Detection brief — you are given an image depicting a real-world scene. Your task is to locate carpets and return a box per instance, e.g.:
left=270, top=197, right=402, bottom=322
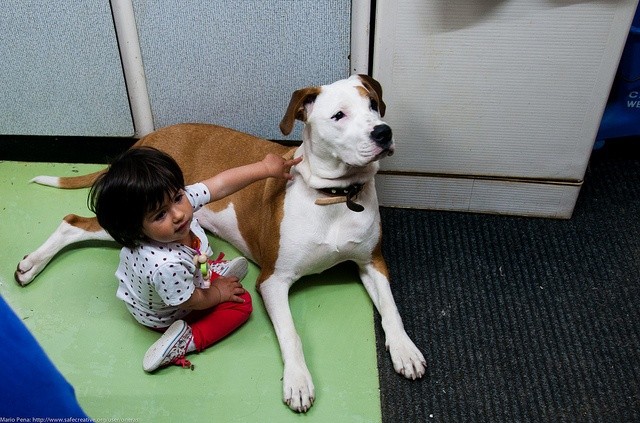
left=373, top=134, right=640, bottom=423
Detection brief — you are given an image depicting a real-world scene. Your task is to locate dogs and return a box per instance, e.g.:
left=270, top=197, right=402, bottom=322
left=12, top=72, right=431, bottom=416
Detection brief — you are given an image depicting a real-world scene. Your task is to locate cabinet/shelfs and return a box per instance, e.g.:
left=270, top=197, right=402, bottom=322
left=363, top=0, right=638, bottom=222
left=0, top=0, right=359, bottom=153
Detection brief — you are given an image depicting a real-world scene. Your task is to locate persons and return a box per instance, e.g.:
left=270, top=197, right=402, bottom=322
left=86, top=144, right=305, bottom=371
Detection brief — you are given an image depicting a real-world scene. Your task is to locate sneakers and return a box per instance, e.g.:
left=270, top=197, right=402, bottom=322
left=208, top=252, right=249, bottom=283
left=143, top=320, right=193, bottom=372
